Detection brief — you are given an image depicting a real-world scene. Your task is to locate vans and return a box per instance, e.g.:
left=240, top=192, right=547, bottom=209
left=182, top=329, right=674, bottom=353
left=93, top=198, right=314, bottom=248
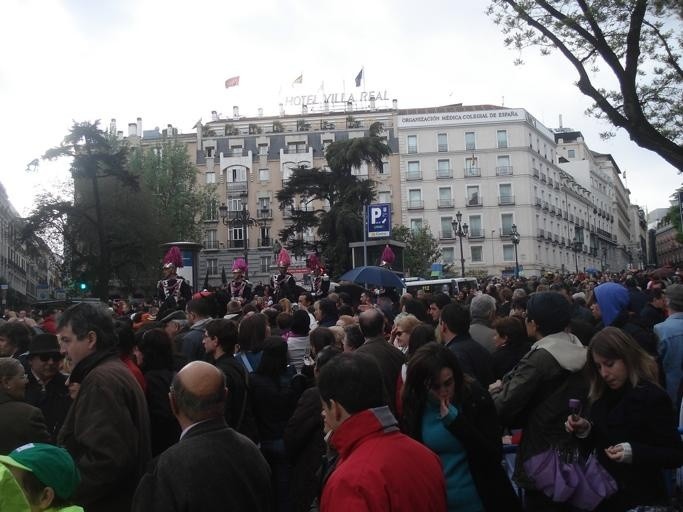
left=402, top=278, right=479, bottom=302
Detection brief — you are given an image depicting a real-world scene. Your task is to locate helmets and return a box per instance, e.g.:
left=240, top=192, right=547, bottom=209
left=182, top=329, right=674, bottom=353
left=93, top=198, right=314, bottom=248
left=379, top=261, right=392, bottom=268
left=162, top=261, right=176, bottom=275
left=277, top=261, right=288, bottom=270
left=232, top=267, right=244, bottom=275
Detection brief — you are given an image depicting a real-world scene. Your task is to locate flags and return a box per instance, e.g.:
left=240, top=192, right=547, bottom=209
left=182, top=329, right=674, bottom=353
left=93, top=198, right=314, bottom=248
left=292, top=75, right=302, bottom=84
left=354, top=69, right=362, bottom=88
left=224, top=76, right=239, bottom=89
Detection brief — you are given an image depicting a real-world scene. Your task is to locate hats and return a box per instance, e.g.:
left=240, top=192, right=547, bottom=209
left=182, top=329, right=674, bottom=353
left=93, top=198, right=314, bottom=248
left=156, top=309, right=188, bottom=326
left=139, top=328, right=176, bottom=368
left=24, top=334, right=63, bottom=364
left=0, top=442, right=83, bottom=502
left=661, top=283, right=683, bottom=306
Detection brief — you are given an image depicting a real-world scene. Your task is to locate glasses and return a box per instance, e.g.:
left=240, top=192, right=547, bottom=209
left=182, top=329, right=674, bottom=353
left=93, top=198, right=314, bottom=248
left=5, top=372, right=29, bottom=381
left=393, top=327, right=409, bottom=338
left=33, top=351, right=65, bottom=364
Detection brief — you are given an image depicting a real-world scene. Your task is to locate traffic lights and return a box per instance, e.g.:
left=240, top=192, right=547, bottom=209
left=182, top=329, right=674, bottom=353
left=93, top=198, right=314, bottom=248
left=80, top=272, right=88, bottom=292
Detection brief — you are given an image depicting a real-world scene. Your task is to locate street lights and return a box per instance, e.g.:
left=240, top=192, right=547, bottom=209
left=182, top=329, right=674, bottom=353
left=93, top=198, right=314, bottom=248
left=570, top=238, right=582, bottom=275
left=219, top=190, right=269, bottom=280
left=452, top=210, right=469, bottom=278
left=491, top=228, right=495, bottom=275
left=510, top=223, right=520, bottom=278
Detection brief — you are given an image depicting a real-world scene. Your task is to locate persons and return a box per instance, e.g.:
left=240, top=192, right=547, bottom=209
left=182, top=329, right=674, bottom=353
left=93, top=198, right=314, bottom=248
left=2, top=242, right=683, bottom=510
